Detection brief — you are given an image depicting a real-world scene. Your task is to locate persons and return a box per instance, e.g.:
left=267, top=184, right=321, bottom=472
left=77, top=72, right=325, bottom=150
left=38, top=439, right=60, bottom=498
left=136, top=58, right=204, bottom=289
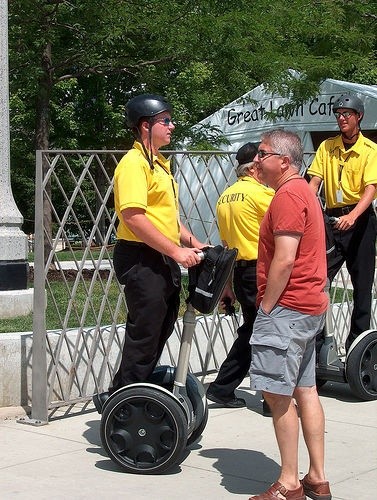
left=247, top=129, right=332, bottom=500
left=205, top=142, right=298, bottom=417
left=308, top=95, right=377, bottom=357
left=109, top=94, right=216, bottom=397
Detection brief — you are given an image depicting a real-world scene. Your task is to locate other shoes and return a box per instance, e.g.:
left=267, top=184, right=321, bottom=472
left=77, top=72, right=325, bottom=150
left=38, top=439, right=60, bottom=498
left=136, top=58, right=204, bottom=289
left=262, top=402, right=301, bottom=417
left=205, top=392, right=246, bottom=408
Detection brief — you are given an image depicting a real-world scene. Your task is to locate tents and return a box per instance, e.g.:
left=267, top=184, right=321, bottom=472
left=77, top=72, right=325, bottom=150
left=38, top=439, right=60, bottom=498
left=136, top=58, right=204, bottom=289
left=166, top=69, right=377, bottom=245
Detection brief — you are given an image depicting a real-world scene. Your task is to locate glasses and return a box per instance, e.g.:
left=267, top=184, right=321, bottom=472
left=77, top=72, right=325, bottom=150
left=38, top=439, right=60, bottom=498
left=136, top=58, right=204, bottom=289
left=334, top=112, right=357, bottom=118
left=255, top=151, right=282, bottom=158
left=153, top=117, right=176, bottom=126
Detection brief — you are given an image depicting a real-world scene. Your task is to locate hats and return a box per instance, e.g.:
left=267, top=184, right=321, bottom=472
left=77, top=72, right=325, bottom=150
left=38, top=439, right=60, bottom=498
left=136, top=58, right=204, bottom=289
left=236, top=142, right=262, bottom=164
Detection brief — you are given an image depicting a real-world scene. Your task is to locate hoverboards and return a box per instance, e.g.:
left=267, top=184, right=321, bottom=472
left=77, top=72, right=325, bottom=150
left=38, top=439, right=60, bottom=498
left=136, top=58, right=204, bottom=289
left=100, top=244, right=240, bottom=475
left=316, top=213, right=377, bottom=401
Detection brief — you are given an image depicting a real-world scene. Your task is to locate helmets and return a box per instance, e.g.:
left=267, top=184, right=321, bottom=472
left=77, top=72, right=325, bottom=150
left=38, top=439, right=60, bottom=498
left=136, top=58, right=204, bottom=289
left=333, top=95, right=364, bottom=114
left=125, top=94, right=174, bottom=127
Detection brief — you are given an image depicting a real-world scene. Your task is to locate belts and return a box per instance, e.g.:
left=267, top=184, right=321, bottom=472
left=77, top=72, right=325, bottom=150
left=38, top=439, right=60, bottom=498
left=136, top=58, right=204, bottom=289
left=325, top=203, right=358, bottom=217
left=234, top=261, right=257, bottom=267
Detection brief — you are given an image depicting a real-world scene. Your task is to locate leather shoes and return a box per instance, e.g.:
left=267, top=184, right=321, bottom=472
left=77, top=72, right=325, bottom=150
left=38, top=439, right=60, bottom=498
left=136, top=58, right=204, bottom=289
left=300, top=473, right=332, bottom=500
left=249, top=481, right=307, bottom=500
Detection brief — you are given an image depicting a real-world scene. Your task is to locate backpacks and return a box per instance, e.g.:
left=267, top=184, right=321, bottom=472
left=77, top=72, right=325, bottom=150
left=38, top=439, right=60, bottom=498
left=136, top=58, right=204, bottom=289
left=185, top=245, right=238, bottom=315
